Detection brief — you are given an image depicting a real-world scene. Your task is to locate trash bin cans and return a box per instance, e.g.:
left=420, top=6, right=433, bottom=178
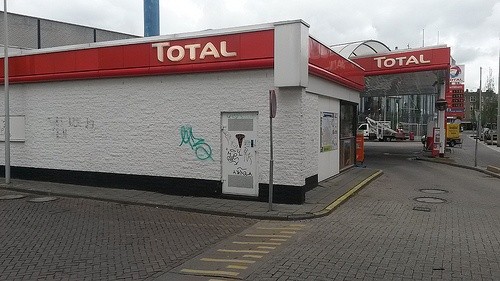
left=409, top=132, right=414, bottom=141
left=426, top=136, right=433, bottom=151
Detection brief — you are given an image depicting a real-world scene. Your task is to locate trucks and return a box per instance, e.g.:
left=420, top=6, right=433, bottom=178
left=358, top=123, right=409, bottom=142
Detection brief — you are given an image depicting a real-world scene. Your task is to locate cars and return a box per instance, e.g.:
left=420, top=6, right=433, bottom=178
left=421, top=126, right=499, bottom=148
